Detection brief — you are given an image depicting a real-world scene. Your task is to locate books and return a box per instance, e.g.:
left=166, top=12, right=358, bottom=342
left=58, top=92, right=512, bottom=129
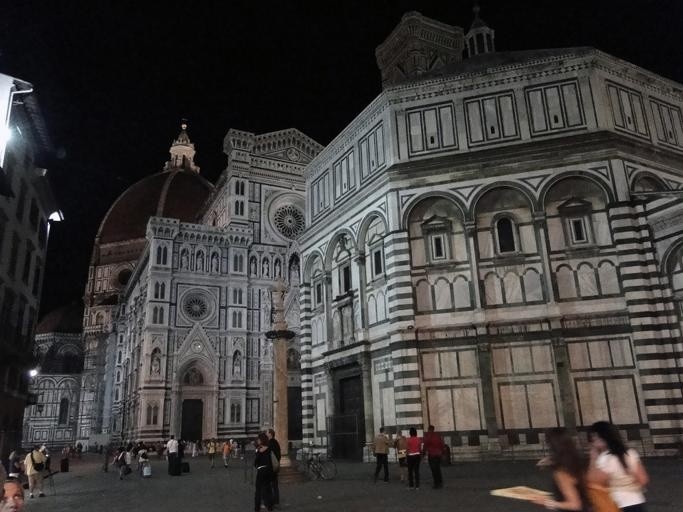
left=489, top=485, right=553, bottom=500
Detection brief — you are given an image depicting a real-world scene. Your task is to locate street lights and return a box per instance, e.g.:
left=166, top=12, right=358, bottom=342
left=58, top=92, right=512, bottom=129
left=33, top=404, right=45, bottom=445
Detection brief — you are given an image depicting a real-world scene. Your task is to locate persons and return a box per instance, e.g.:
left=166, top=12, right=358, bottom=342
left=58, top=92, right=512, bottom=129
left=0, top=427, right=282, bottom=511
left=393, top=429, right=407, bottom=481
left=587, top=421, right=651, bottom=512
left=404, top=426, right=422, bottom=489
left=535, top=425, right=587, bottom=512
left=372, top=428, right=395, bottom=482
left=422, top=424, right=445, bottom=489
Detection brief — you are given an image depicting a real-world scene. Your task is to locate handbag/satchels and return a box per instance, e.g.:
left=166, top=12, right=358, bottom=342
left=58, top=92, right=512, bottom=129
left=269, top=449, right=279, bottom=473
left=31, top=452, right=44, bottom=471
left=584, top=480, right=620, bottom=512
left=141, top=452, right=148, bottom=459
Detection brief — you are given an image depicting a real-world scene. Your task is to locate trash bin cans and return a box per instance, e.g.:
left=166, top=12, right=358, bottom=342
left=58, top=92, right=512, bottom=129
left=61, top=457, right=69, bottom=473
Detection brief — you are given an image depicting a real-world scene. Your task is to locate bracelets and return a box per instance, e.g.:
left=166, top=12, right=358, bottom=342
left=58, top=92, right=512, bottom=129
left=543, top=499, right=555, bottom=509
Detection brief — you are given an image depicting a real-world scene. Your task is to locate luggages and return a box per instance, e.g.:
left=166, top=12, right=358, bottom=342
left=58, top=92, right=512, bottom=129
left=141, top=463, right=152, bottom=478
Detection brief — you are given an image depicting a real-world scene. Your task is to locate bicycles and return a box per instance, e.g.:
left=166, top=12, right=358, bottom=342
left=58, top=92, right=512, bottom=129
left=296, top=452, right=337, bottom=480
